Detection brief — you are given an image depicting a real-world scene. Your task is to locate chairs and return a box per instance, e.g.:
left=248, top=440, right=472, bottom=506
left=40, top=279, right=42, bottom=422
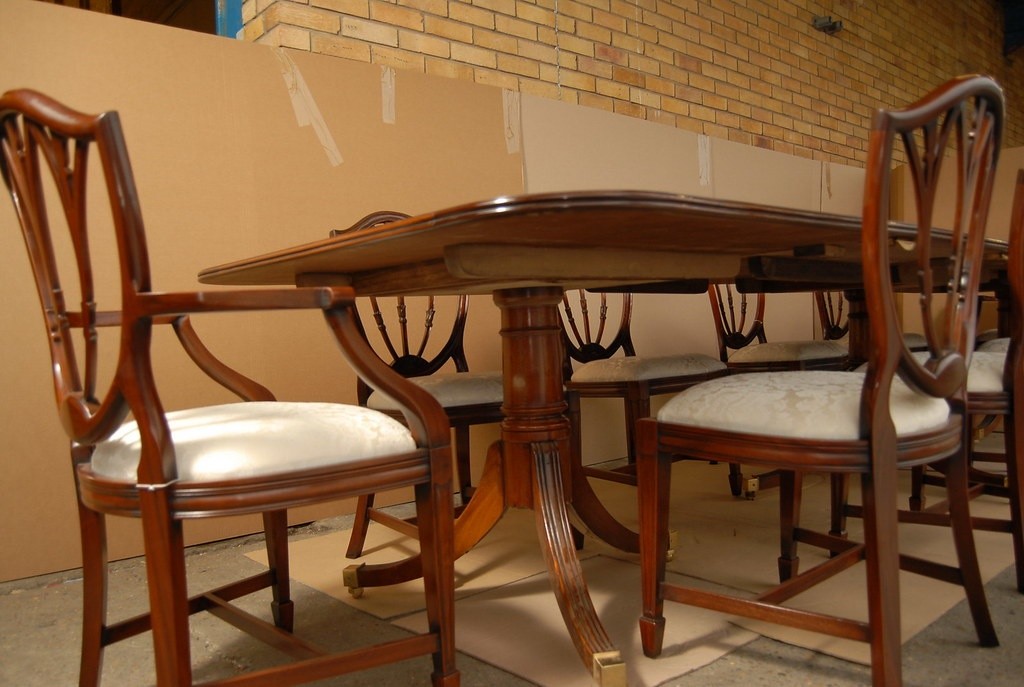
left=638, top=75, right=1006, bottom=686
left=558, top=284, right=743, bottom=495
left=2, top=90, right=463, bottom=686
left=829, top=170, right=1024, bottom=593
left=709, top=281, right=931, bottom=499
left=330, top=211, right=585, bottom=559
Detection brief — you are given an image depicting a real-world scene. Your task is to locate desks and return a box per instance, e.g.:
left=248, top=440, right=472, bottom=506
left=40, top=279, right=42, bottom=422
left=195, top=188, right=1008, bottom=687
